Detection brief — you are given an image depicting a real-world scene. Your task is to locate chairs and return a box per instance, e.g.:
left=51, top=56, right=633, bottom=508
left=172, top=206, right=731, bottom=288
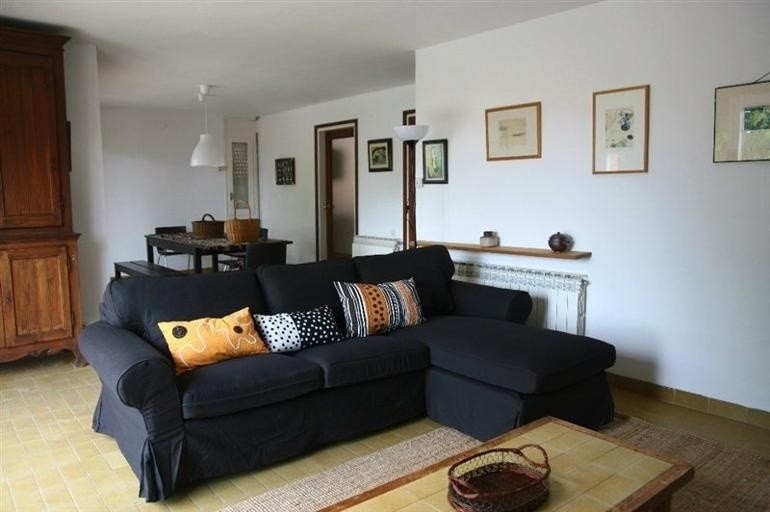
left=217, top=228, right=268, bottom=272
left=155, top=226, right=191, bottom=267
left=245, top=242, right=286, bottom=270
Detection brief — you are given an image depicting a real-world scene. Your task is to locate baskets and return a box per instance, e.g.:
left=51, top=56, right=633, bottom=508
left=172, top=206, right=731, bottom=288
left=191, top=213, right=225, bottom=239
left=225, top=202, right=260, bottom=243
left=447, top=444, right=551, bottom=512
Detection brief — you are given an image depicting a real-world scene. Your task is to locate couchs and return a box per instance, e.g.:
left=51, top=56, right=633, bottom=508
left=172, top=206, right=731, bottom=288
left=77, top=245, right=616, bottom=505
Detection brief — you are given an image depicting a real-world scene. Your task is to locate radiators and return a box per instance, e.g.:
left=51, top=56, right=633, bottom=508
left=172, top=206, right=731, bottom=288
left=350, top=236, right=401, bottom=258
left=450, top=261, right=589, bottom=337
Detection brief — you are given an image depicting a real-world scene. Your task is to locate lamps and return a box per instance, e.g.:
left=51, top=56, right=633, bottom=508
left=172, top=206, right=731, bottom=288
left=189, top=84, right=225, bottom=169
left=392, top=124, right=430, bottom=250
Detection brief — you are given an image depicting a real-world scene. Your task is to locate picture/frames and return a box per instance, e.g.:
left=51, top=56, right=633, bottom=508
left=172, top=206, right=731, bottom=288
left=713, top=80, right=770, bottom=163
left=592, top=84, right=650, bottom=175
left=485, top=101, right=542, bottom=161
left=367, top=137, right=393, bottom=172
left=422, top=139, right=448, bottom=184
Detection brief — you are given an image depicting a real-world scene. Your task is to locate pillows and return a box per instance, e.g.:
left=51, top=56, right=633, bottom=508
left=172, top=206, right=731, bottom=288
left=252, top=302, right=345, bottom=355
left=155, top=304, right=272, bottom=377
left=330, top=276, right=428, bottom=341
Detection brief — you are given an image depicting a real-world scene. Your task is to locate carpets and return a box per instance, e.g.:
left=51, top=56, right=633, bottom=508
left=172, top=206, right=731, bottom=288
left=214, top=408, right=770, bottom=512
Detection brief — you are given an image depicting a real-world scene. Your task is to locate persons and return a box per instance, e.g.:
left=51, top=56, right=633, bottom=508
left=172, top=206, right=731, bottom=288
left=372, top=147, right=386, bottom=165
left=427, top=146, right=443, bottom=179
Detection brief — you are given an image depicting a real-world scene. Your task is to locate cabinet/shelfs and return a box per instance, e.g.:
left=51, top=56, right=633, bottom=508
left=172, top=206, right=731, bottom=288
left=0, top=233, right=88, bottom=366
left=0, top=23, right=71, bottom=230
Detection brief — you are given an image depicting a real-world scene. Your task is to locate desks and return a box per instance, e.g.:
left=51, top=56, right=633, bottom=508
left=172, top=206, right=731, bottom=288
left=144, top=232, right=294, bottom=273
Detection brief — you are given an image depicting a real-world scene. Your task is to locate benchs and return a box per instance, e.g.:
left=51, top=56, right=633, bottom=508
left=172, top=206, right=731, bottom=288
left=112, top=260, right=189, bottom=279
